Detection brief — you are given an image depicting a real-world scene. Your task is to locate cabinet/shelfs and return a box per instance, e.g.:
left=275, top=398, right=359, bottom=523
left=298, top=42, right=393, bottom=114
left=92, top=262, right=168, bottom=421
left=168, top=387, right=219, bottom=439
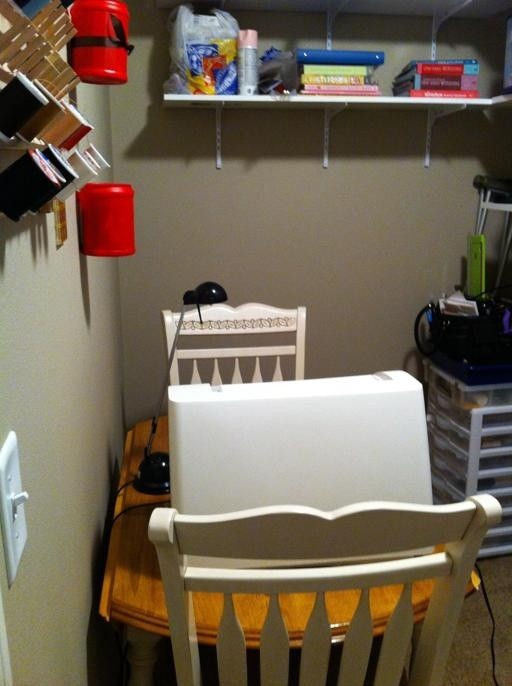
left=162, top=0, right=512, bottom=168
left=422, top=358, right=512, bottom=559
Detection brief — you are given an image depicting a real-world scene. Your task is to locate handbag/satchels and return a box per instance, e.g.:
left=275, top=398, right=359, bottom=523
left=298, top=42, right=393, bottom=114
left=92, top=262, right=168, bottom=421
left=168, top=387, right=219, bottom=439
left=414, top=287, right=511, bottom=365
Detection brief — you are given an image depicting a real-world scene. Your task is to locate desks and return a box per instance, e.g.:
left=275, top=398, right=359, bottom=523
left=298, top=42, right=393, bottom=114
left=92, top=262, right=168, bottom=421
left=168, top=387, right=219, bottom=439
left=99, top=415, right=475, bottom=686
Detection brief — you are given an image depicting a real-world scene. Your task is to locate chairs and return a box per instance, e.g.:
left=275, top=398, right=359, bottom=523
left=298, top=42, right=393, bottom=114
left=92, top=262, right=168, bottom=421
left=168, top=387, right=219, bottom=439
left=160, top=302, right=306, bottom=385
left=149, top=494, right=502, bottom=686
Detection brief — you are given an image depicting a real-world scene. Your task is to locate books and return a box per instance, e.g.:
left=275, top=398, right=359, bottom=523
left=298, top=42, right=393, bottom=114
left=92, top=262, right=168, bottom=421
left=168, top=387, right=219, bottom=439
left=392, top=58, right=480, bottom=97
left=443, top=297, right=478, bottom=315
left=294, top=48, right=385, bottom=96
